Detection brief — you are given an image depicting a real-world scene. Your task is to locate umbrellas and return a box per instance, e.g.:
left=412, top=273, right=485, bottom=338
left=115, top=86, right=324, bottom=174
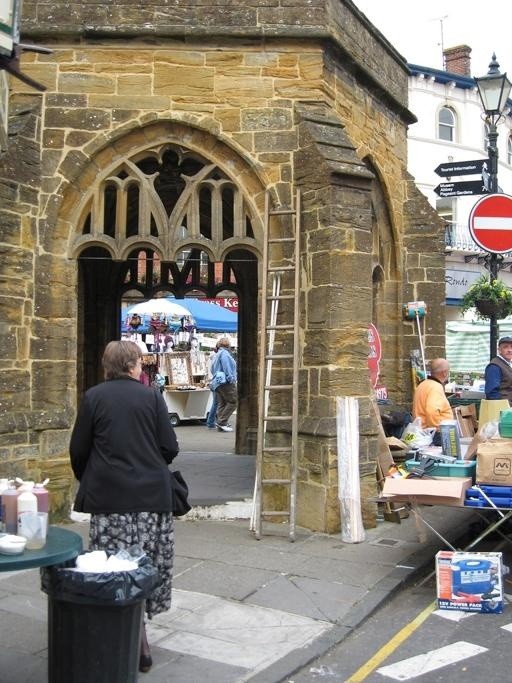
left=127, top=299, right=192, bottom=317
left=121, top=297, right=238, bottom=341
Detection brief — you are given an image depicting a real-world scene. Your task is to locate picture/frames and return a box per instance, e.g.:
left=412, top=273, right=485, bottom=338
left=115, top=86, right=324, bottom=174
left=166, top=350, right=195, bottom=385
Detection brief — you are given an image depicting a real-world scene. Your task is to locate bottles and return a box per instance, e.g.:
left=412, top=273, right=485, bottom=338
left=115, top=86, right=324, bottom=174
left=0, top=476, right=51, bottom=536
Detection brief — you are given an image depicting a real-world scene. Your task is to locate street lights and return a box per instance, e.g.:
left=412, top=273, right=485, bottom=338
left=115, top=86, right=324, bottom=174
left=471, top=48, right=512, bottom=359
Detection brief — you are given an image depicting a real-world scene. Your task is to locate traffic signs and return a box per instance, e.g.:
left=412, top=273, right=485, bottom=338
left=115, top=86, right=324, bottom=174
left=433, top=158, right=489, bottom=174
left=433, top=180, right=487, bottom=196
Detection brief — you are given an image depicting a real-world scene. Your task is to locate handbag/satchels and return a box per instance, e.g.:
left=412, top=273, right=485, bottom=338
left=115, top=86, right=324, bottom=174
left=173, top=471, right=191, bottom=516
left=215, top=370, right=227, bottom=384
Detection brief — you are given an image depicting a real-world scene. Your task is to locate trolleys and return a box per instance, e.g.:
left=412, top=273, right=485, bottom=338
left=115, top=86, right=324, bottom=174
left=159, top=386, right=215, bottom=427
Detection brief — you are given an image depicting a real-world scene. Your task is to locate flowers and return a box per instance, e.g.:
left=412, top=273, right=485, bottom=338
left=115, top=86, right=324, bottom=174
left=458, top=273, right=512, bottom=320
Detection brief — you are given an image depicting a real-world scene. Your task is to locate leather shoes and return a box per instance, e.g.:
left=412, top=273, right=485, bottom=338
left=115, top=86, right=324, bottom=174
left=140, top=655, right=152, bottom=672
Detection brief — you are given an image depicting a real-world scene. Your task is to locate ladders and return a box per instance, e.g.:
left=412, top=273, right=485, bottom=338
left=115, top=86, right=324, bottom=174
left=254, top=188, right=300, bottom=540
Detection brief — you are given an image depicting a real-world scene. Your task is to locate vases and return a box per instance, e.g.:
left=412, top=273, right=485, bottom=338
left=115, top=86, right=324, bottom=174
left=474, top=295, right=506, bottom=315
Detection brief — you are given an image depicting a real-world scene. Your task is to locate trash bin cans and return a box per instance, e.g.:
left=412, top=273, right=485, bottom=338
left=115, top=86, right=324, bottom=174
left=39, top=550, right=154, bottom=683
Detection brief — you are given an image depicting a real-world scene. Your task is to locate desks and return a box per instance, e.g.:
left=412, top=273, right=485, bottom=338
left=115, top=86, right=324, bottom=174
left=0, top=521, right=83, bottom=576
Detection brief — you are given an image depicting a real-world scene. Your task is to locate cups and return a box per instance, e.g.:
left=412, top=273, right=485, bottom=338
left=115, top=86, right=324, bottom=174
left=1, top=534, right=26, bottom=555
left=200, top=380, right=205, bottom=387
left=19, top=511, right=46, bottom=550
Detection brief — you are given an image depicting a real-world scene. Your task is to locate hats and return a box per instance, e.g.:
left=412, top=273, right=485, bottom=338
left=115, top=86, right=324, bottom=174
left=499, top=337, right=512, bottom=344
left=189, top=337, right=198, bottom=348
left=164, top=336, right=173, bottom=345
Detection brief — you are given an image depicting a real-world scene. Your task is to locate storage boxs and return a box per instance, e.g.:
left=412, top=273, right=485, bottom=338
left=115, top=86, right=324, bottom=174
left=379, top=474, right=475, bottom=508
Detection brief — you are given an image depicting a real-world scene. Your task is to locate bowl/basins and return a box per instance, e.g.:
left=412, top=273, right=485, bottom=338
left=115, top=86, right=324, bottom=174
left=164, top=385, right=178, bottom=389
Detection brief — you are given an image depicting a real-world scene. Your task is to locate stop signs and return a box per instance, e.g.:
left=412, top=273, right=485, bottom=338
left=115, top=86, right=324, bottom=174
left=468, top=194, right=511, bottom=255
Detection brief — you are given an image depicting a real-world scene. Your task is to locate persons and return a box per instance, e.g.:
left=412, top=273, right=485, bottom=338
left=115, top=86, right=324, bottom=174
left=164, top=335, right=175, bottom=353
left=207, top=341, right=222, bottom=429
left=483, top=336, right=511, bottom=407
left=210, top=337, right=238, bottom=432
left=69, top=339, right=181, bottom=673
left=189, top=335, right=200, bottom=352
left=413, top=356, right=454, bottom=445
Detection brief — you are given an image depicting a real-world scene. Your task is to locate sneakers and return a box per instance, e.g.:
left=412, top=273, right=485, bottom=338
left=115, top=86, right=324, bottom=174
left=208, top=423, right=234, bottom=432
